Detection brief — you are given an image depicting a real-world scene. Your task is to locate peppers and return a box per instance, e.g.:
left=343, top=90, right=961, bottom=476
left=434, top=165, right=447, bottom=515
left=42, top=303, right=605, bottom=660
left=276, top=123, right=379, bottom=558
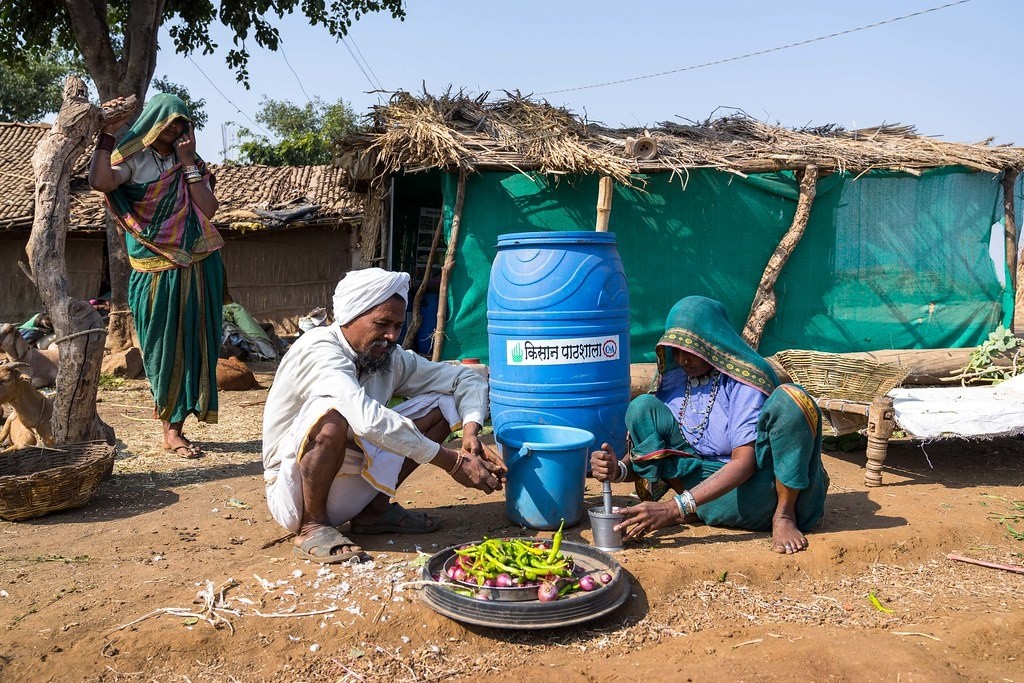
left=450, top=518, right=580, bottom=599
left=718, top=570, right=727, bottom=583
left=869, top=592, right=896, bottom=613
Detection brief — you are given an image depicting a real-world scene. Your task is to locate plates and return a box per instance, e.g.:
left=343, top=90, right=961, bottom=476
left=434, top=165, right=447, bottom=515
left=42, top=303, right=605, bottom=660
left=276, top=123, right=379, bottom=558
left=422, top=537, right=630, bottom=629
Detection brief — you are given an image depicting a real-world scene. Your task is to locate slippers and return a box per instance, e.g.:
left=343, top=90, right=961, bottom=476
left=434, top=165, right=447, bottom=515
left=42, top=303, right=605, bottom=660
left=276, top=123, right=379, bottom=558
left=293, top=526, right=364, bottom=563
left=350, top=502, right=441, bottom=534
left=164, top=443, right=202, bottom=459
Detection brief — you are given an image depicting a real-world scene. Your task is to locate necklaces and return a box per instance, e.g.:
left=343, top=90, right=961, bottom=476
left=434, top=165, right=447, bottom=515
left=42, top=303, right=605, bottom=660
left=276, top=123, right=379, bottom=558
left=678, top=370, right=720, bottom=445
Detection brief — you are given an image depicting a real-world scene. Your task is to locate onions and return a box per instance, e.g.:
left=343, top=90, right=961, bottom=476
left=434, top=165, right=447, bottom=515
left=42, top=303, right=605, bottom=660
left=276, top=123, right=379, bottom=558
left=430, top=537, right=612, bottom=602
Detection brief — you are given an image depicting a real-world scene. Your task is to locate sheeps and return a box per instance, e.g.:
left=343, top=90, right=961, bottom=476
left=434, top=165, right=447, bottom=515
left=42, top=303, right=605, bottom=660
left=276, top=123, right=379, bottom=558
left=214, top=355, right=269, bottom=390
left=0, top=322, right=60, bottom=453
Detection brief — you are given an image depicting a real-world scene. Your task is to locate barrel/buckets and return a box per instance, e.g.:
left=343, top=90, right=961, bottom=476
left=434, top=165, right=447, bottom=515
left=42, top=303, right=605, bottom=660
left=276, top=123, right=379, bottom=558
left=486, top=232, right=631, bottom=530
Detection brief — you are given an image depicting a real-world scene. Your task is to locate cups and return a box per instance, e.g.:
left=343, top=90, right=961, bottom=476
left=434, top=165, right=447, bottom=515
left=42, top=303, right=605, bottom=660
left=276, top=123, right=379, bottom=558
left=588, top=506, right=626, bottom=551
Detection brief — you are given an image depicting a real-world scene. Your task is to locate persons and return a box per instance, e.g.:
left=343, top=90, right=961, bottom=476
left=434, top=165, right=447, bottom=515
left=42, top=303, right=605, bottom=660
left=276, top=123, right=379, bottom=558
left=17, top=312, right=51, bottom=343
left=263, top=267, right=509, bottom=564
left=88, top=92, right=234, bottom=458
left=589, top=295, right=830, bottom=555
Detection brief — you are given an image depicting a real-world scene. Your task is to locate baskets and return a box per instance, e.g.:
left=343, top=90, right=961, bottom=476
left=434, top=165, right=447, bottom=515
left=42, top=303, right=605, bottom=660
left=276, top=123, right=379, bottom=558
left=0, top=439, right=118, bottom=521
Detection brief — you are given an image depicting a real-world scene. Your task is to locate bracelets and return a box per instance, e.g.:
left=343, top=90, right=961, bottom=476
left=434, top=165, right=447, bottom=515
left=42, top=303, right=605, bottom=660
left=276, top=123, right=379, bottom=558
left=95, top=131, right=115, bottom=154
left=614, top=461, right=628, bottom=483
left=183, top=165, right=202, bottom=184
left=675, top=489, right=696, bottom=519
left=446, top=450, right=463, bottom=475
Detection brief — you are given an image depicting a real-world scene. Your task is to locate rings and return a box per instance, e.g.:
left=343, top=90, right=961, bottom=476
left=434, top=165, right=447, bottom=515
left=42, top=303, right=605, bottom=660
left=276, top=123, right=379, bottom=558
left=179, top=138, right=184, bottom=142
